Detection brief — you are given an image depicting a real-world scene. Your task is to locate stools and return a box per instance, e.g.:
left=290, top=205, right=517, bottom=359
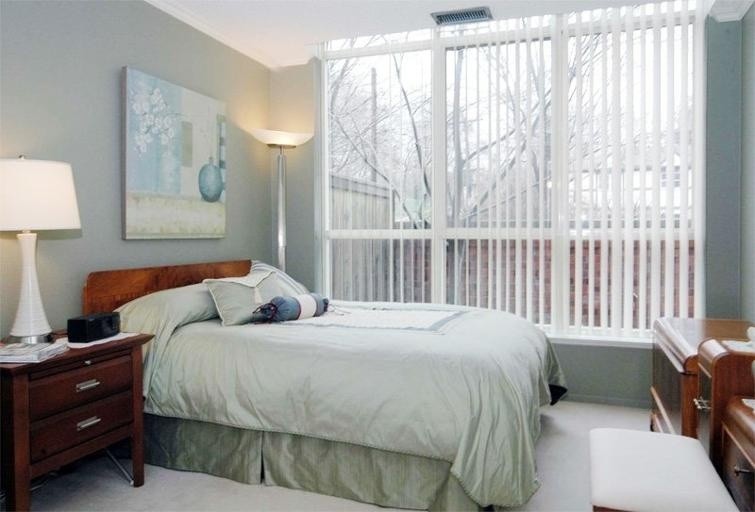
left=588, top=429, right=739, bottom=512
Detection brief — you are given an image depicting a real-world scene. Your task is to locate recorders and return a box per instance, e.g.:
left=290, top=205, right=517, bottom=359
left=66, top=311, right=120, bottom=343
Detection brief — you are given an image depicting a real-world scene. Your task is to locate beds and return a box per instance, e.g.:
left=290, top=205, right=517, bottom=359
left=84, top=260, right=555, bottom=512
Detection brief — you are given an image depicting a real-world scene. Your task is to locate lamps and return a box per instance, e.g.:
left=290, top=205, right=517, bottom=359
left=1, top=155, right=83, bottom=344
left=246, top=127, right=314, bottom=273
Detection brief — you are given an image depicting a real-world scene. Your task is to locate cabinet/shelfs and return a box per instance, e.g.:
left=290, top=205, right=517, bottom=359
left=0, top=328, right=155, bottom=511
left=650, top=317, right=755, bottom=511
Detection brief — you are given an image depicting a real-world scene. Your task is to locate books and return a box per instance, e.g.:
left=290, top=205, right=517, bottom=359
left=0, top=343, right=68, bottom=364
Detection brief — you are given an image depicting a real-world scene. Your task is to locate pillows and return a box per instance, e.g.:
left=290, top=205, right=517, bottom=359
left=201, top=271, right=328, bottom=325
